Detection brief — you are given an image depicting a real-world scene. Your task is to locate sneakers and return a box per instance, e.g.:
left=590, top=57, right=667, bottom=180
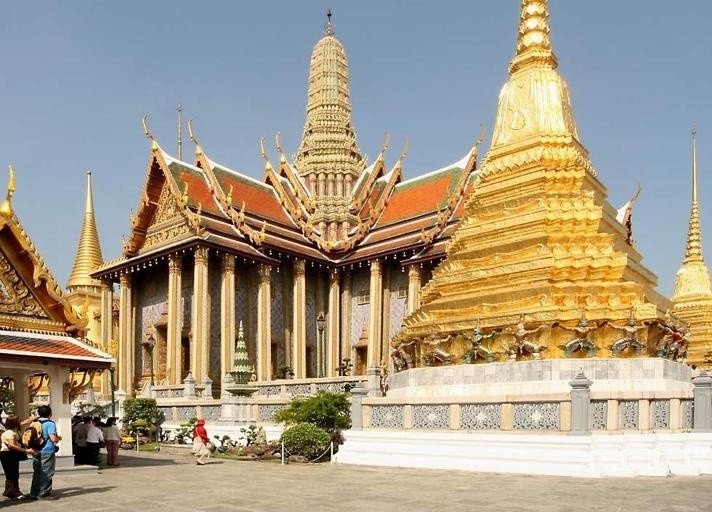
left=38, top=492, right=59, bottom=501
left=195, top=459, right=204, bottom=464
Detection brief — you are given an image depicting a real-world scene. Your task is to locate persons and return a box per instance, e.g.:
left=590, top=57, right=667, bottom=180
left=376, top=356, right=391, bottom=398
left=460, top=328, right=496, bottom=363
left=668, top=318, right=687, bottom=358
left=391, top=335, right=415, bottom=370
left=608, top=318, right=646, bottom=348
left=505, top=322, right=548, bottom=357
left=0, top=414, right=41, bottom=500
left=553, top=314, right=608, bottom=354
left=656, top=312, right=678, bottom=356
left=676, top=320, right=693, bottom=358
left=71, top=414, right=124, bottom=469
left=22, top=404, right=63, bottom=502
left=414, top=333, right=452, bottom=365
left=190, top=418, right=212, bottom=465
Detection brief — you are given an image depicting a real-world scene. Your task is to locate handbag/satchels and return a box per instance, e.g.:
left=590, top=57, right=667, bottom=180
left=206, top=443, right=213, bottom=451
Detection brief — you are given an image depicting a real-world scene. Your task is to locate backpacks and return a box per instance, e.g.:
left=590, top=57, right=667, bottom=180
left=22, top=419, right=52, bottom=451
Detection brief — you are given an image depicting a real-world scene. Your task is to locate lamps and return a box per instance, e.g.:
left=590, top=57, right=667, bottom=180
left=316, top=311, right=326, bottom=334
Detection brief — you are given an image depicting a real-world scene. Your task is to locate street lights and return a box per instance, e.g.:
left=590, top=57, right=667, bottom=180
left=147, top=334, right=156, bottom=385
left=107, top=358, right=116, bottom=418
left=316, top=313, right=328, bottom=377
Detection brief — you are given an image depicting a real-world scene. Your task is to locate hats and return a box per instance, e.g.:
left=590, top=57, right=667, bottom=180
left=197, top=419, right=205, bottom=425
